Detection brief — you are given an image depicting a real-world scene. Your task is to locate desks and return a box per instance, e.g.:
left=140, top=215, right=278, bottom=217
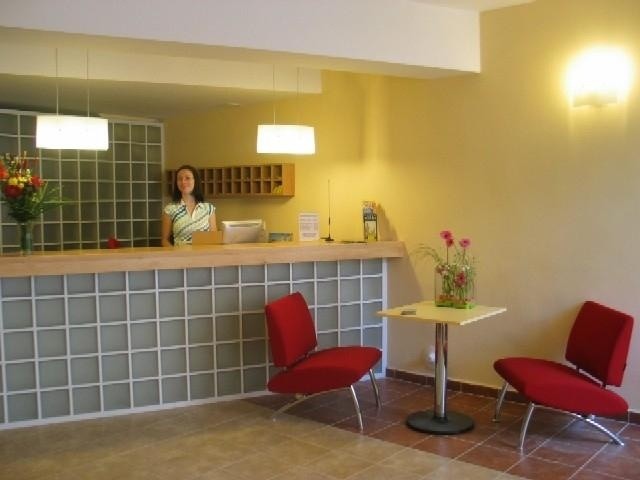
left=377, top=299, right=507, bottom=437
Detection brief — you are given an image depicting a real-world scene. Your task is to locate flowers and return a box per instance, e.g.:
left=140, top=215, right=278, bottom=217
left=455, top=238, right=485, bottom=271
left=0, top=151, right=70, bottom=249
left=418, top=229, right=455, bottom=268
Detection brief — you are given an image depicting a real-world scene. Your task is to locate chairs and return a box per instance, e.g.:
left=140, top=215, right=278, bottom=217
left=261, top=291, right=380, bottom=434
left=490, top=302, right=635, bottom=452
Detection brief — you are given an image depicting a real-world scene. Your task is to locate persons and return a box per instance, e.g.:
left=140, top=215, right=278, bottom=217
left=160, top=164, right=218, bottom=247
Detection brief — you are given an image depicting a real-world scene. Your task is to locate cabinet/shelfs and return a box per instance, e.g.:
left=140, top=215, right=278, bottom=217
left=165, top=162, right=294, bottom=198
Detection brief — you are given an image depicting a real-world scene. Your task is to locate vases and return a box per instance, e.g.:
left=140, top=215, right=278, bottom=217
left=454, top=271, right=476, bottom=309
left=18, top=217, right=35, bottom=255
left=434, top=270, right=454, bottom=307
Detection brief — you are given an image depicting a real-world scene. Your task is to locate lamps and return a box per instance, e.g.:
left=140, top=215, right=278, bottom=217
left=35, top=46, right=109, bottom=152
left=255, top=63, right=317, bottom=155
